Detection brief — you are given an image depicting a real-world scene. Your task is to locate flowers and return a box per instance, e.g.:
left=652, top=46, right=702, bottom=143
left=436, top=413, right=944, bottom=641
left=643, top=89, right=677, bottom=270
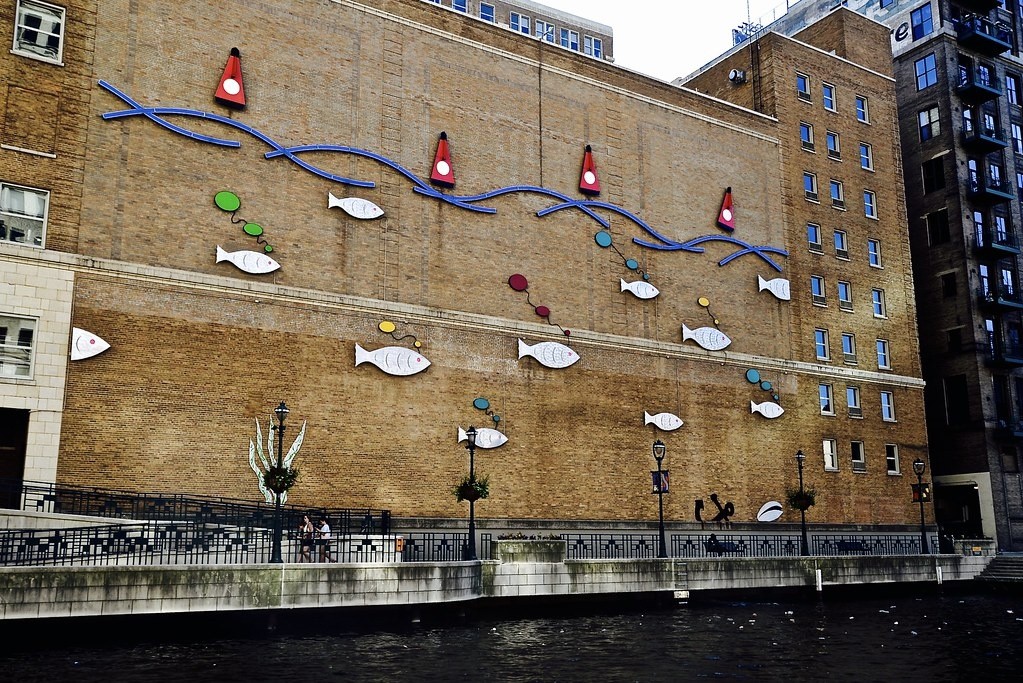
left=785, top=481, right=816, bottom=511
left=454, top=470, right=490, bottom=503
left=262, top=465, right=299, bottom=493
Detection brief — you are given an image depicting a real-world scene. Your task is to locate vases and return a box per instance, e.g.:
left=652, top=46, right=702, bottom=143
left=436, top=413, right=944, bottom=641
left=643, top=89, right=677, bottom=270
left=463, top=488, right=482, bottom=502
left=793, top=498, right=811, bottom=511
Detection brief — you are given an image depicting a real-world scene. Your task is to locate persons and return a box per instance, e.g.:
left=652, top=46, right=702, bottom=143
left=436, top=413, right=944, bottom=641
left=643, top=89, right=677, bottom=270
left=297, top=515, right=314, bottom=563
left=708, top=534, right=724, bottom=557
left=315, top=517, right=336, bottom=563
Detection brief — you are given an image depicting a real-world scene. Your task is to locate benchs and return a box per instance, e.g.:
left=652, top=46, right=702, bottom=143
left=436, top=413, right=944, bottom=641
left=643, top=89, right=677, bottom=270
left=836, top=542, right=872, bottom=555
left=704, top=541, right=743, bottom=557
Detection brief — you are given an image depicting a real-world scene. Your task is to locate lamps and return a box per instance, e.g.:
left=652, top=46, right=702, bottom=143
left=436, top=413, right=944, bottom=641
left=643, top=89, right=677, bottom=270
left=729, top=68, right=747, bottom=85
